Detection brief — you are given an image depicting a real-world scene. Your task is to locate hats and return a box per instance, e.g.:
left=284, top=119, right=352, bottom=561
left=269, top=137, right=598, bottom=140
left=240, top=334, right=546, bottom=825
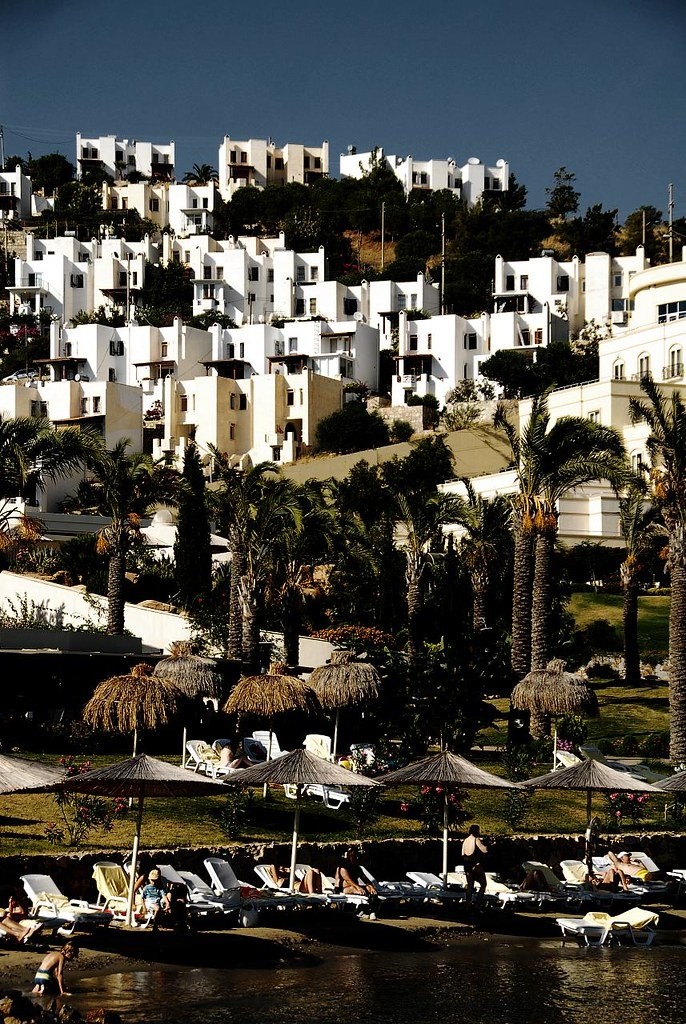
left=148, top=870, right=158, bottom=879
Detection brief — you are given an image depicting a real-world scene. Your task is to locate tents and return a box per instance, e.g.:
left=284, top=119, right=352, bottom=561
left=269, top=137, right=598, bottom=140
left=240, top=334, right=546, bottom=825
left=62, top=753, right=237, bottom=933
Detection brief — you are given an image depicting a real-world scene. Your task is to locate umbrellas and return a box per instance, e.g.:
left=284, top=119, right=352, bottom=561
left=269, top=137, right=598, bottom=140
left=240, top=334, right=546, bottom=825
left=224, top=747, right=385, bottom=897
left=519, top=758, right=668, bottom=881
left=650, top=768, right=686, bottom=796
left=375, top=749, right=527, bottom=891
left=510, top=658, right=600, bottom=774
left=84, top=640, right=381, bottom=807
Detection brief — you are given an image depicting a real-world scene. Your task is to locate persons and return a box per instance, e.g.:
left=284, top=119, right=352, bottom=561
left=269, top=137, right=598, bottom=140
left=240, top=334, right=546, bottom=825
left=218, top=738, right=272, bottom=769
left=278, top=846, right=378, bottom=900
left=607, top=850, right=686, bottom=888
left=0, top=879, right=34, bottom=944
left=460, top=825, right=497, bottom=909
left=131, top=852, right=173, bottom=933
left=31, top=940, right=80, bottom=996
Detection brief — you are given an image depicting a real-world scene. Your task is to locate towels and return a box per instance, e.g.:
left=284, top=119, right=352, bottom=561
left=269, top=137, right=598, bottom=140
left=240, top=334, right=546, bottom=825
left=583, top=906, right=660, bottom=930
left=303, top=735, right=331, bottom=761
left=92, top=865, right=130, bottom=901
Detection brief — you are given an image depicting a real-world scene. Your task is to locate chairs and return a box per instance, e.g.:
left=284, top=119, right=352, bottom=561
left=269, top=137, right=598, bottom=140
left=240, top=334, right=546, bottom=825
left=184, top=731, right=383, bottom=807
left=550, top=747, right=649, bottom=781
left=20, top=851, right=686, bottom=948
